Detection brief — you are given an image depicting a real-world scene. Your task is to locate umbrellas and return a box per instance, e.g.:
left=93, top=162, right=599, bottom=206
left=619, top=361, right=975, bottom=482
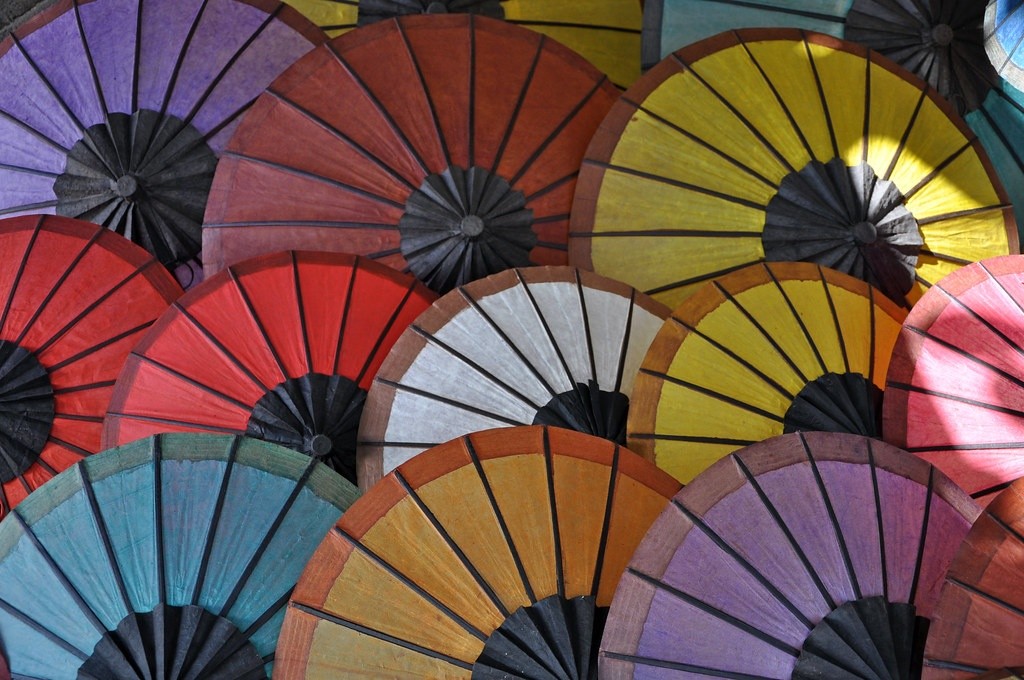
left=921, top=477, right=1024, bottom=680
left=627, top=262, right=909, bottom=484
left=641, top=0, right=1024, bottom=249
left=567, top=28, right=1020, bottom=314
left=285, top=0, right=642, bottom=94
left=0, top=431, right=362, bottom=680
left=101, top=249, right=438, bottom=486
left=0, top=213, right=185, bottom=521
left=355, top=264, right=671, bottom=492
left=597, top=432, right=983, bottom=680
left=203, top=12, right=617, bottom=293
left=883, top=254, right=1024, bottom=509
left=271, top=425, right=683, bottom=680
left=983, top=0, right=1024, bottom=92
left=0, top=0, right=330, bottom=290
